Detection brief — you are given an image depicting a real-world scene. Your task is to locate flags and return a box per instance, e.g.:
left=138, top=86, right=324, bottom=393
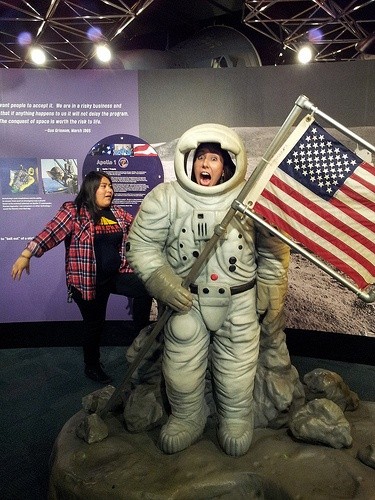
left=243, top=113, right=375, bottom=290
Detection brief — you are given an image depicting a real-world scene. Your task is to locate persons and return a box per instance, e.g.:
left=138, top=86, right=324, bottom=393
left=11, top=170, right=154, bottom=384
left=191, top=140, right=235, bottom=189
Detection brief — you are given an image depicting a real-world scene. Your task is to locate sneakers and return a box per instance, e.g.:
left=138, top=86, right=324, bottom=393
left=85, top=366, right=112, bottom=383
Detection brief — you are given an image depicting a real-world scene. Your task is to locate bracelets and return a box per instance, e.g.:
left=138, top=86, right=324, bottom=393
left=20, top=254, right=30, bottom=260
